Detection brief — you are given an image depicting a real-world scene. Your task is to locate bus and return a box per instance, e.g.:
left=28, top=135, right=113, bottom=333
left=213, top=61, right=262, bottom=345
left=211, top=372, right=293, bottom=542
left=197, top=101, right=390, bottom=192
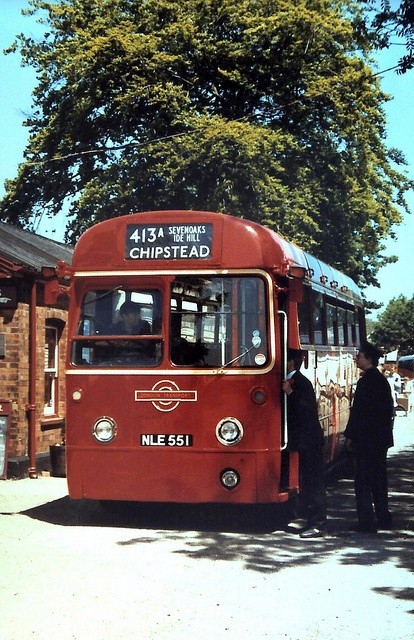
left=54, top=209, right=370, bottom=502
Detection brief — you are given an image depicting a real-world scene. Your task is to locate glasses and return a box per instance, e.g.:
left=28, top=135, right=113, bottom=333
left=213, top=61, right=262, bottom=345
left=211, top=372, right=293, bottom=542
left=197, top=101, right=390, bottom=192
left=356, top=355, right=360, bottom=358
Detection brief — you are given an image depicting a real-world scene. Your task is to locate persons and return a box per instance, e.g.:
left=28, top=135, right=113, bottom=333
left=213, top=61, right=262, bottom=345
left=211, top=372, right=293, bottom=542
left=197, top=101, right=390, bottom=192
left=390, top=361, right=400, bottom=417
left=381, top=369, right=390, bottom=378
left=284, top=350, right=335, bottom=537
left=408, top=375, right=413, bottom=422
left=96, top=301, right=155, bottom=365
left=343, top=343, right=394, bottom=532
left=404, top=377, right=407, bottom=389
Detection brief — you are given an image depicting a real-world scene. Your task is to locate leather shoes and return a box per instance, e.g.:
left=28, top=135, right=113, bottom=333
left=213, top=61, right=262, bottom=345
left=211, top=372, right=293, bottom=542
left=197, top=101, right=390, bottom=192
left=300, top=529, right=324, bottom=538
left=378, top=523, right=392, bottom=530
left=348, top=525, right=376, bottom=534
left=287, top=519, right=308, bottom=534
